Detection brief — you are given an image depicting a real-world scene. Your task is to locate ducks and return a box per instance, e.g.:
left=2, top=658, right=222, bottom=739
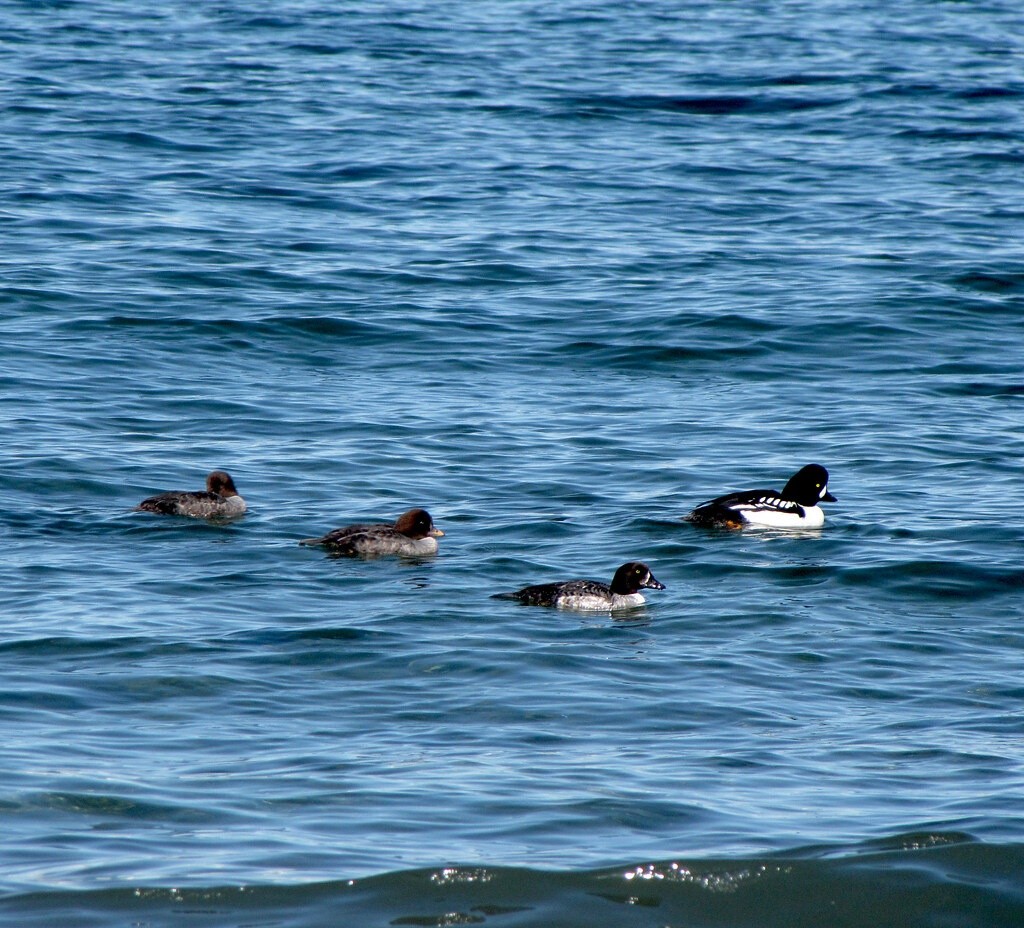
left=490, top=562, right=665, bottom=612
left=135, top=471, right=246, bottom=518
left=299, top=509, right=445, bottom=556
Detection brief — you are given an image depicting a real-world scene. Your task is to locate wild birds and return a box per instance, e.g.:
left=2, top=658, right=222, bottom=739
left=683, top=464, right=838, bottom=528
